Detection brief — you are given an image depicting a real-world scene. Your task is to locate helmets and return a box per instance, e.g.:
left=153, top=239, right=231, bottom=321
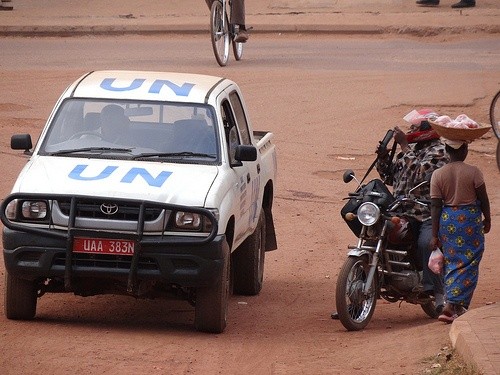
left=403, top=109, right=441, bottom=143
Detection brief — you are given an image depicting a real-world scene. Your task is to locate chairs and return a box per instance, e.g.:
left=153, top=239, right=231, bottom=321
left=83, top=112, right=100, bottom=137
left=173, top=119, right=207, bottom=153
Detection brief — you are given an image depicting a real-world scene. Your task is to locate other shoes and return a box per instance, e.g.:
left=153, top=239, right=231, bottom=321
left=215, top=20, right=223, bottom=41
left=451, top=0, right=476, bottom=7
left=331, top=303, right=364, bottom=319
left=416, top=0, right=439, bottom=4
left=434, top=294, right=445, bottom=312
left=235, top=30, right=248, bottom=43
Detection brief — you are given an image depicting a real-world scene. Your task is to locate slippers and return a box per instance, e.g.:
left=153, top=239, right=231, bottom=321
left=438, top=314, right=459, bottom=323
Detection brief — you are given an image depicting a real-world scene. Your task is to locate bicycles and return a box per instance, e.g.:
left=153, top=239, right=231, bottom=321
left=209, top=0, right=253, bottom=66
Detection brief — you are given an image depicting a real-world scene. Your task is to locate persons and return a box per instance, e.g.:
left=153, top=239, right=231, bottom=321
left=205, top=0, right=249, bottom=43
left=416, top=0, right=476, bottom=9
left=81, top=105, right=131, bottom=150
left=429, top=140, right=493, bottom=322
left=331, top=106, right=451, bottom=319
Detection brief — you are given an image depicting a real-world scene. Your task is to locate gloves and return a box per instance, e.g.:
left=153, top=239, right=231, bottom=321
left=394, top=126, right=408, bottom=147
left=374, top=140, right=392, bottom=157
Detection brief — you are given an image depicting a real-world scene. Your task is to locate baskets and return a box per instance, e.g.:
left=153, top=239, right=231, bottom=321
left=428, top=117, right=492, bottom=141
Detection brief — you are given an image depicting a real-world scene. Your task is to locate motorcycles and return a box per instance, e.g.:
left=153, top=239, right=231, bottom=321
left=335, top=171, right=436, bottom=330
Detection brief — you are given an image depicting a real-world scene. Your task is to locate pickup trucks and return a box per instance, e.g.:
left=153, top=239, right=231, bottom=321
left=0, top=70, right=278, bottom=333
